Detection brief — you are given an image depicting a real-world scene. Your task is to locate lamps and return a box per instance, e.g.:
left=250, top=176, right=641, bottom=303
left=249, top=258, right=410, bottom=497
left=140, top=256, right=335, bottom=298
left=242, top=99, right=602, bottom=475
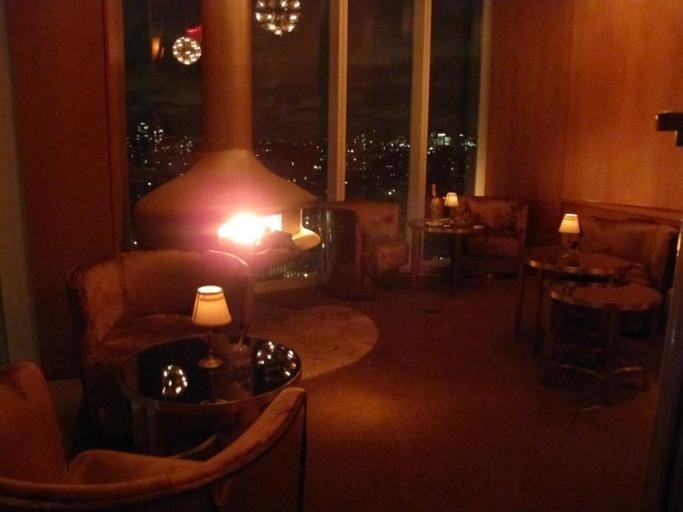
left=191, top=284, right=231, bottom=369
left=444, top=192, right=458, bottom=207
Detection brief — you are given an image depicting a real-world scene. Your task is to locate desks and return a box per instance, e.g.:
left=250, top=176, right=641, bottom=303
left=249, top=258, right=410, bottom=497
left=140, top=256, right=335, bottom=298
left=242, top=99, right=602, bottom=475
left=117, top=334, right=301, bottom=458
left=542, top=281, right=662, bottom=404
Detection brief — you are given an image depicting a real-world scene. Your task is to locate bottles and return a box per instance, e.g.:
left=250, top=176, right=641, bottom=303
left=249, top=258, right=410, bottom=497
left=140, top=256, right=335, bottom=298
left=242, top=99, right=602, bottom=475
left=430, top=182, right=442, bottom=224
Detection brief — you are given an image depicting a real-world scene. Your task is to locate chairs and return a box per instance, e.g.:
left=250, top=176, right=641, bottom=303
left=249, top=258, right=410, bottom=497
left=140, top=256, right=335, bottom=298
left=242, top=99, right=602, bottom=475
left=0, top=362, right=307, bottom=512
left=65, top=251, right=254, bottom=449
